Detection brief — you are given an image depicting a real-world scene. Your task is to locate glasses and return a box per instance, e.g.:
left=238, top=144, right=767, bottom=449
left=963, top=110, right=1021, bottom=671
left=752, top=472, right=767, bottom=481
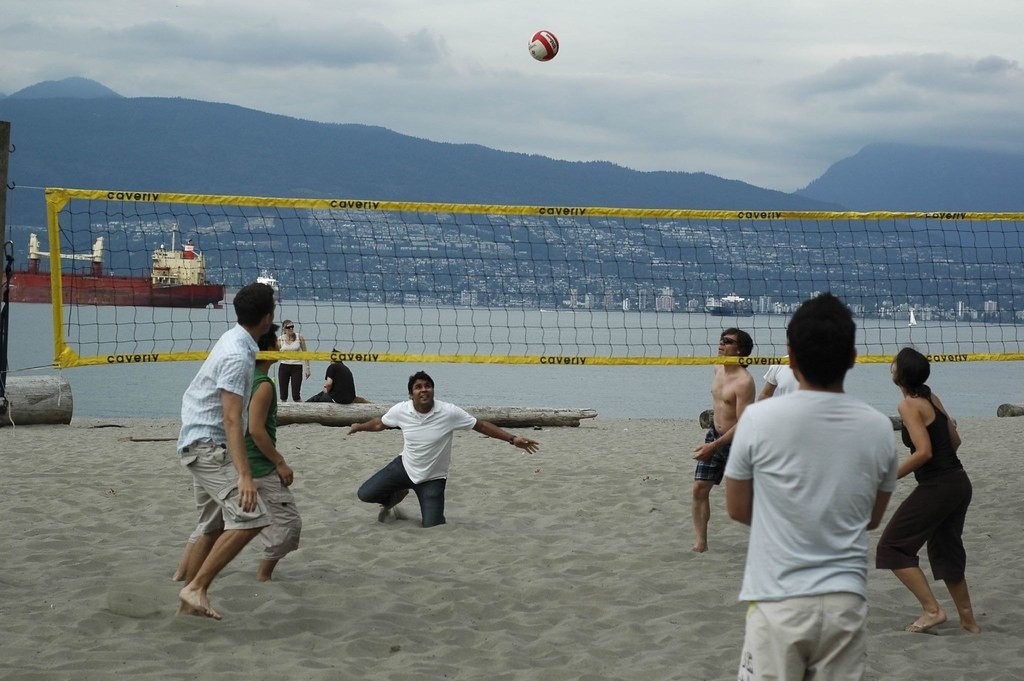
left=285, top=325, right=294, bottom=329
left=720, top=337, right=737, bottom=344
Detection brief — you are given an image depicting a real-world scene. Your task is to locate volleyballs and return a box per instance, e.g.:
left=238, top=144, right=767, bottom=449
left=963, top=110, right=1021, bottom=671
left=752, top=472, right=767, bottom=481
left=528, top=30, right=560, bottom=63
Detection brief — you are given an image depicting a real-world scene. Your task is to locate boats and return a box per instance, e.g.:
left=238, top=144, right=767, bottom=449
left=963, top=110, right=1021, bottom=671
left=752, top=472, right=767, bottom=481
left=257, top=269, right=285, bottom=303
left=0, top=221, right=227, bottom=309
left=705, top=293, right=755, bottom=317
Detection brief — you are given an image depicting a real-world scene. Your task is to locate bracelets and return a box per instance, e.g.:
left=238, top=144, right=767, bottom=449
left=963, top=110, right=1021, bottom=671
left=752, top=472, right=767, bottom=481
left=510, top=433, right=517, bottom=444
left=306, top=366, right=311, bottom=368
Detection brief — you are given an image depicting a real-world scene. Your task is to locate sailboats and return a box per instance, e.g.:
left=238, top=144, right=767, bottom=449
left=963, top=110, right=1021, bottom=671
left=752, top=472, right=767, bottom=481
left=908, top=309, right=917, bottom=326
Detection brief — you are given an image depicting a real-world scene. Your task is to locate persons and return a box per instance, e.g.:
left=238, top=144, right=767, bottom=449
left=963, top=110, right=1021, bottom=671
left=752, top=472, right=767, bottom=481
left=277, top=319, right=311, bottom=402
left=304, top=349, right=356, bottom=404
left=172, top=322, right=301, bottom=583
left=757, top=353, right=800, bottom=400
left=174, top=281, right=275, bottom=619
left=725, top=291, right=899, bottom=681
left=875, top=346, right=982, bottom=635
left=691, top=328, right=756, bottom=553
left=347, top=371, right=540, bottom=527
